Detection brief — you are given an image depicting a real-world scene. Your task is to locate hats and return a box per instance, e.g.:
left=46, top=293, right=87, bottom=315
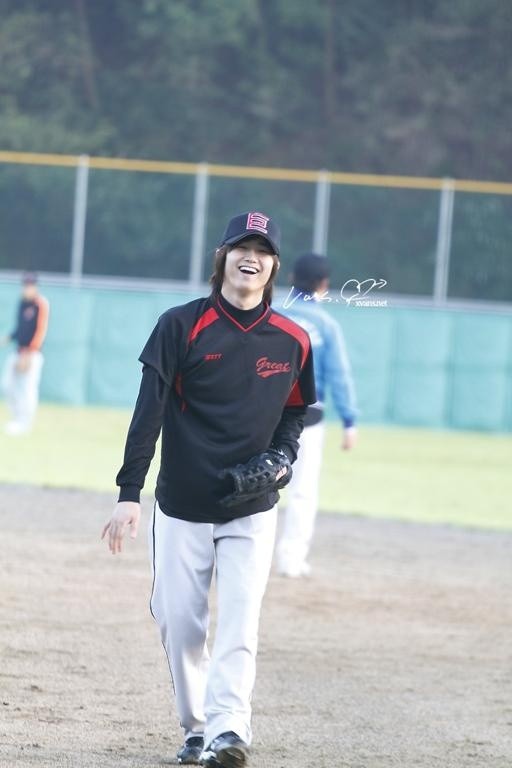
left=294, top=253, right=334, bottom=280
left=220, top=211, right=281, bottom=257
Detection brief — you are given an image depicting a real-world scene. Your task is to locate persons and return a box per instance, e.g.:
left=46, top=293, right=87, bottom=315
left=269, top=252, right=360, bottom=582
left=0, top=268, right=52, bottom=438
left=97, top=211, right=319, bottom=768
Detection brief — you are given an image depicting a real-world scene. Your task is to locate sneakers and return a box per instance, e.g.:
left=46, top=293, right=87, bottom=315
left=176, top=736, right=205, bottom=765
left=278, top=560, right=311, bottom=578
left=198, top=731, right=250, bottom=768
